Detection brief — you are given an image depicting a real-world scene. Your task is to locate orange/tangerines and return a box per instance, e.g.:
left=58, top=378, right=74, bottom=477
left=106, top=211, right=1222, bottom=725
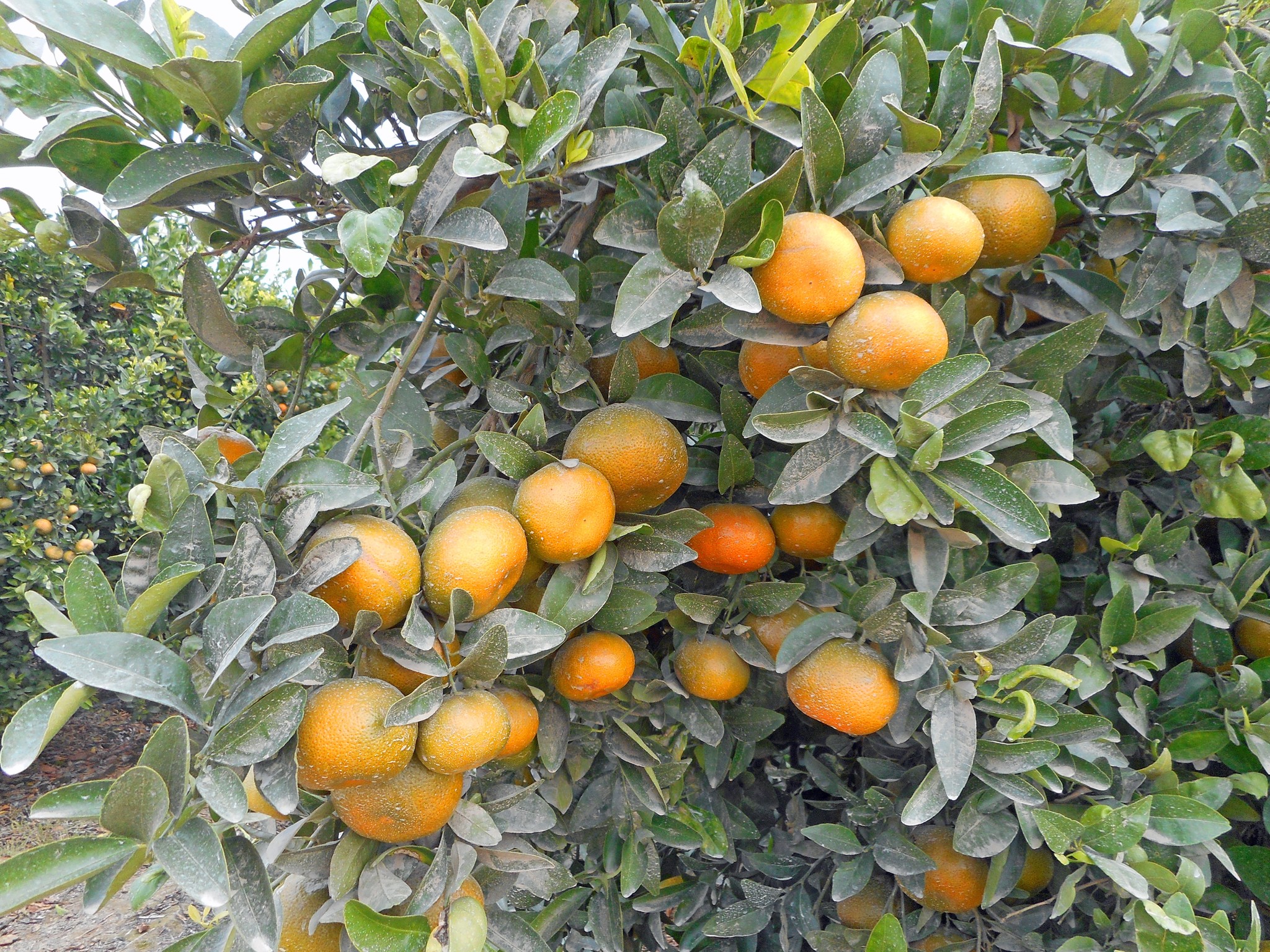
left=246, top=176, right=1270, bottom=952
left=4, top=218, right=343, bottom=563
